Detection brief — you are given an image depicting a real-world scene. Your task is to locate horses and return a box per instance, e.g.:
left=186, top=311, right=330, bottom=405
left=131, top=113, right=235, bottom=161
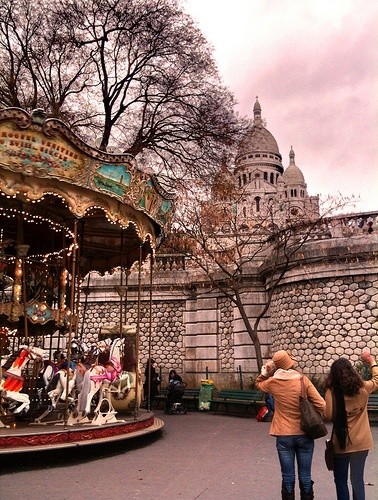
left=0, top=336, right=127, bottom=415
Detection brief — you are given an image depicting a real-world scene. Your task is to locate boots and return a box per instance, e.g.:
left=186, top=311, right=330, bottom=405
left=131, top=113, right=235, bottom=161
left=281, top=480, right=296, bottom=500
left=300, top=480, right=315, bottom=500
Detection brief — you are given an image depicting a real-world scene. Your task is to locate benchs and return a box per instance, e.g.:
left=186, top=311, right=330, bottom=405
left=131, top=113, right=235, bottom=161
left=367, top=392, right=378, bottom=412
left=154, top=387, right=201, bottom=410
left=207, top=388, right=264, bottom=416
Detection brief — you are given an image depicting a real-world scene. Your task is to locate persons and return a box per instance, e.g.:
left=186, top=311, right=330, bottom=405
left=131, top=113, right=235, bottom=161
left=165, top=370, right=184, bottom=413
left=255, top=350, right=327, bottom=500
left=144, top=360, right=161, bottom=411
left=325, top=351, right=378, bottom=500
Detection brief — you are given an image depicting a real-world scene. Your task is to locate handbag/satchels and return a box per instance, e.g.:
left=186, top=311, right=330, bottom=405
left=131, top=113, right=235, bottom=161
left=325, top=442, right=335, bottom=471
left=300, top=376, right=328, bottom=440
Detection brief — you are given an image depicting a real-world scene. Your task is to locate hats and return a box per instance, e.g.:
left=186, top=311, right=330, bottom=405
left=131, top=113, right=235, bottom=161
left=273, top=351, right=295, bottom=371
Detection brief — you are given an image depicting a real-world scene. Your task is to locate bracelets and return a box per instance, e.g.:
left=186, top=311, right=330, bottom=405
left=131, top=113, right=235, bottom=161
left=371, top=364, right=378, bottom=369
